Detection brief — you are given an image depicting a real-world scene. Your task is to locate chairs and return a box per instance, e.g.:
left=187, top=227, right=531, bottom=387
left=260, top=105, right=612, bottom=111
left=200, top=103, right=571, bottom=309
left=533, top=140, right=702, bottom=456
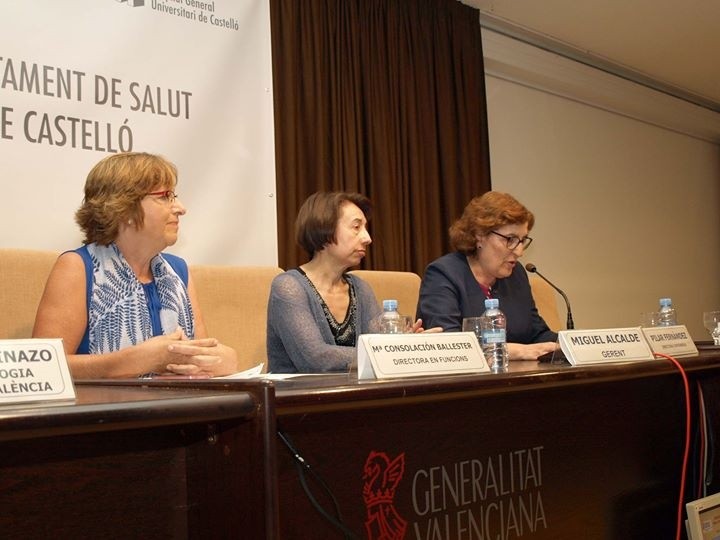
left=0, top=248, right=566, bottom=375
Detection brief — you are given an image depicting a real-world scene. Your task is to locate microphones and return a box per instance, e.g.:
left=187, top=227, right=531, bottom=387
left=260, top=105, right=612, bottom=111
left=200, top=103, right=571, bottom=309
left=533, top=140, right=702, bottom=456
left=525, top=262, right=575, bottom=362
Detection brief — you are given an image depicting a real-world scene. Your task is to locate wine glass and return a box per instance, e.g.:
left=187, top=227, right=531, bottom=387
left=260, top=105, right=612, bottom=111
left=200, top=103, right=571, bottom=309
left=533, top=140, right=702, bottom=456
left=703, top=311, right=720, bottom=346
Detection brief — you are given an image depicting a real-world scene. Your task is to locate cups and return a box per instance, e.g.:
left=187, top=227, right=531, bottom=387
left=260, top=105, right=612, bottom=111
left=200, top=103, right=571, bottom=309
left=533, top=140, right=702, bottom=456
left=367, top=315, right=414, bottom=334
left=461, top=317, right=482, bottom=347
left=640, top=311, right=658, bottom=328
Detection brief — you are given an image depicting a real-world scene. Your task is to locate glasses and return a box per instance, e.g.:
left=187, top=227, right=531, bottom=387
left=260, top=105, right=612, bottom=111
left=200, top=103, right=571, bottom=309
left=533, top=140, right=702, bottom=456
left=145, top=189, right=178, bottom=205
left=490, top=229, right=533, bottom=251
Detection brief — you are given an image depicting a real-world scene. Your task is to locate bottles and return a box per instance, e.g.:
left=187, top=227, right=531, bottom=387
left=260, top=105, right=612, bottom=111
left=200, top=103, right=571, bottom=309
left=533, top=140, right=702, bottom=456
left=480, top=299, right=509, bottom=369
left=377, top=300, right=404, bottom=334
left=656, top=298, right=677, bottom=326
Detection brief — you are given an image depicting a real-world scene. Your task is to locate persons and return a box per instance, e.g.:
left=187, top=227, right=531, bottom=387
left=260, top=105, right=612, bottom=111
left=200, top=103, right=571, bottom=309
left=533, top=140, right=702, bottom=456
left=415, top=191, right=561, bottom=363
left=266, top=191, right=444, bottom=375
left=31, top=153, right=241, bottom=378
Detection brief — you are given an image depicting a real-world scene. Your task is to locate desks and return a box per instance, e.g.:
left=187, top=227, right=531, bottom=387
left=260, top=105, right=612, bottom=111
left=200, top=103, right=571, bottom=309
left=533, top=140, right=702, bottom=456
left=0, top=344, right=720, bottom=540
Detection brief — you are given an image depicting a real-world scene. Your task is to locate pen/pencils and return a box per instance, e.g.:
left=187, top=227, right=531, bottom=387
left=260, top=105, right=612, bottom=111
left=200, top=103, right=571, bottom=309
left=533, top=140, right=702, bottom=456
left=138, top=374, right=209, bottom=380
